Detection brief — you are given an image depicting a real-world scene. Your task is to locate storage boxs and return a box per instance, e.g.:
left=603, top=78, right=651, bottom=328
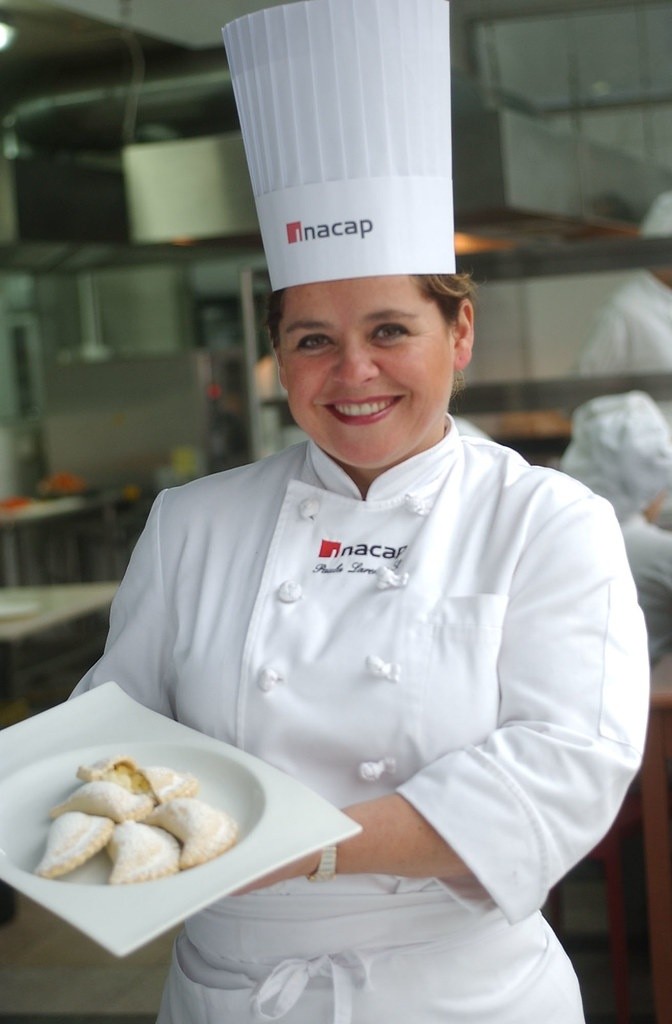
left=120, top=130, right=260, bottom=246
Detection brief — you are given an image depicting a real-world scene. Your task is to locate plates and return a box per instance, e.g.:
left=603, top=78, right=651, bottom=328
left=1, top=682, right=363, bottom=956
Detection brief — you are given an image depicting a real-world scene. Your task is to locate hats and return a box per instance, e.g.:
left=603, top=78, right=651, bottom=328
left=562, top=394, right=672, bottom=518
left=221, top=0, right=458, bottom=293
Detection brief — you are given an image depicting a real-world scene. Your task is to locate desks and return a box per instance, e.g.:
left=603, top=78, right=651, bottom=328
left=0, top=582, right=120, bottom=715
left=636, top=648, right=672, bottom=1024
left=0, top=483, right=130, bottom=582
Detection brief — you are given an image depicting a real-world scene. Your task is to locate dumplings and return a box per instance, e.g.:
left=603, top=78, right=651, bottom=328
left=35, top=757, right=237, bottom=884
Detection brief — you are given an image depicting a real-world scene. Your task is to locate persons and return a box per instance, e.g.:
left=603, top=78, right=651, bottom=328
left=67, top=0, right=651, bottom=1024
left=596, top=192, right=672, bottom=530
left=557, top=388, right=672, bottom=666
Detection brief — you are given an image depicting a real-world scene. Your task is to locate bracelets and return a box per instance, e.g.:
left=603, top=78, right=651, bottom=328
left=307, top=844, right=337, bottom=882
